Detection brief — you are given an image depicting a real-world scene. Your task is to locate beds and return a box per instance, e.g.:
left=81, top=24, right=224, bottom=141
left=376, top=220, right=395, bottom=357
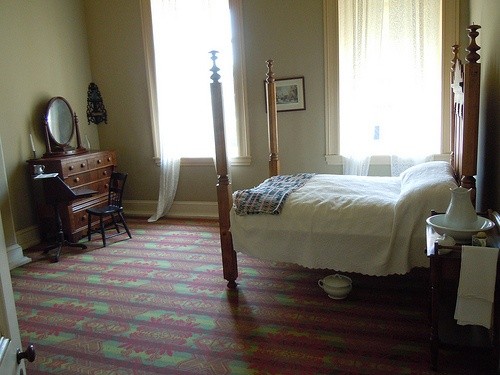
left=208, top=21, right=481, bottom=292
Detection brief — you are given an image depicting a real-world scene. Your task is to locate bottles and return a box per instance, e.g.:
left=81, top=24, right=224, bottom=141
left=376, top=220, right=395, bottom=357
left=443, top=185, right=481, bottom=230
left=83, top=134, right=91, bottom=152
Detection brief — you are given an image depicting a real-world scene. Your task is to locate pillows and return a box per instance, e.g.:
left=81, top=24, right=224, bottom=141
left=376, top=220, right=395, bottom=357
left=396, top=161, right=458, bottom=212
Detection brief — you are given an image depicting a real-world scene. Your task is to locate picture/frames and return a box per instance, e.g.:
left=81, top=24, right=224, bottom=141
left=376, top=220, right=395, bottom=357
left=264, top=77, right=306, bottom=113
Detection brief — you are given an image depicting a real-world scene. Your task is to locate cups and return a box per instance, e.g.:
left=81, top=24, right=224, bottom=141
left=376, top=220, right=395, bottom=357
left=471, top=234, right=487, bottom=247
left=318, top=273, right=353, bottom=300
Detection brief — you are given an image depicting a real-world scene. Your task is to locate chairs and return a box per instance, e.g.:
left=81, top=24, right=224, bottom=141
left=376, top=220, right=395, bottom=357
left=30, top=174, right=99, bottom=262
left=86, top=173, right=132, bottom=247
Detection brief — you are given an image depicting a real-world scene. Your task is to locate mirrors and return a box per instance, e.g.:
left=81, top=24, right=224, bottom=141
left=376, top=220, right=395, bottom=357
left=41, top=96, right=88, bottom=159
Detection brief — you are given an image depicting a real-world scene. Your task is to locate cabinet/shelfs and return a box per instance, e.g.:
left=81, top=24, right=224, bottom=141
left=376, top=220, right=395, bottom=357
left=25, top=150, right=122, bottom=243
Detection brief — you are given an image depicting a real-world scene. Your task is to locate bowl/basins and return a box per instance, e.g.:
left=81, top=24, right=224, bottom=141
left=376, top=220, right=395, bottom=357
left=425, top=213, right=496, bottom=240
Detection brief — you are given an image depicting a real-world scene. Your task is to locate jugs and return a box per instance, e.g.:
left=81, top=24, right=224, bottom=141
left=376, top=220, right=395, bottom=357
left=33, top=164, right=45, bottom=176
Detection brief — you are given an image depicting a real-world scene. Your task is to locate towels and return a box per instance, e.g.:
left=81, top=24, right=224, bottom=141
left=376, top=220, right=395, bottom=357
left=453, top=245, right=499, bottom=330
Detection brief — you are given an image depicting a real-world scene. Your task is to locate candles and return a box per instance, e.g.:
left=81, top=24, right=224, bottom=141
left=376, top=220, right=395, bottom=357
left=30, top=134, right=35, bottom=151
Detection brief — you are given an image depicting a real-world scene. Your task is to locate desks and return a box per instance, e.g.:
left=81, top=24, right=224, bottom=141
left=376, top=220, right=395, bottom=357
left=422, top=209, right=500, bottom=370
